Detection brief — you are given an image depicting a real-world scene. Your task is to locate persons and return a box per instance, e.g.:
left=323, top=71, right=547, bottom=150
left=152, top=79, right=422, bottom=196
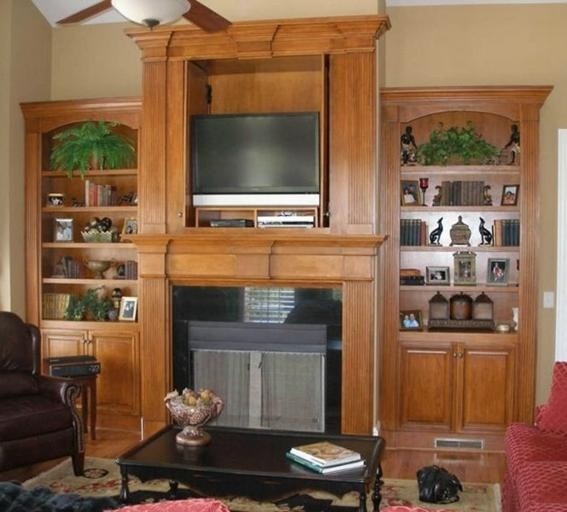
left=403, top=185, right=417, bottom=203
left=410, top=313, right=419, bottom=327
left=401, top=126, right=417, bottom=163
left=503, top=124, right=520, bottom=163
left=403, top=314, right=411, bottom=328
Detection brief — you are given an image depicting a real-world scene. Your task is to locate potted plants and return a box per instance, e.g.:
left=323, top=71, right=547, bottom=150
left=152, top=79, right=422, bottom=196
left=415, top=127, right=500, bottom=165
left=47, top=119, right=135, bottom=178
left=64, top=288, right=115, bottom=321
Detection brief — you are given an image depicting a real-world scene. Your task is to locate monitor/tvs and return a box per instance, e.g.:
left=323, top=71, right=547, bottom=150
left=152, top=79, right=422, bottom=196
left=190, top=111, right=320, bottom=206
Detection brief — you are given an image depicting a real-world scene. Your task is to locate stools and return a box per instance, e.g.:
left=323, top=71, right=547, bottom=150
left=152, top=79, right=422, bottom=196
left=44, top=356, right=98, bottom=441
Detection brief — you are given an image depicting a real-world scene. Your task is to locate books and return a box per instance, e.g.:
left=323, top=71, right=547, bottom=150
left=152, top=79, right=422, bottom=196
left=43, top=293, right=79, bottom=319
left=400, top=219, right=428, bottom=246
left=492, top=219, right=520, bottom=246
left=439, top=180, right=484, bottom=206
left=285, top=440, right=365, bottom=474
left=85, top=179, right=117, bottom=207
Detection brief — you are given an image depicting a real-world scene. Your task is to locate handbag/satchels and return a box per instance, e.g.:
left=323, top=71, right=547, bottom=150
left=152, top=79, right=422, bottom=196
left=415, top=465, right=463, bottom=504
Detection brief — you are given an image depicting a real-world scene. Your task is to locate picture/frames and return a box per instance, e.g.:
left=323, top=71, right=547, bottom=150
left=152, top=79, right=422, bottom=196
left=399, top=180, right=422, bottom=205
left=452, top=252, right=478, bottom=286
left=500, top=184, right=518, bottom=205
left=118, top=296, right=137, bottom=321
left=400, top=309, right=425, bottom=331
left=119, top=215, right=138, bottom=240
left=52, top=216, right=74, bottom=243
left=425, top=265, right=450, bottom=285
left=45, top=192, right=66, bottom=208
left=485, top=257, right=510, bottom=287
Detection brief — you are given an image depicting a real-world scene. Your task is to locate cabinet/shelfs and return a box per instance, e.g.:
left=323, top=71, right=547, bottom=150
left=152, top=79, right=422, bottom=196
left=379, top=82, right=554, bottom=437
left=121, top=14, right=392, bottom=434
left=19, top=97, right=142, bottom=430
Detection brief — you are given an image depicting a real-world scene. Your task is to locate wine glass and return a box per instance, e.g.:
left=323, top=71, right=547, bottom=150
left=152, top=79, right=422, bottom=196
left=419, top=177, right=427, bottom=205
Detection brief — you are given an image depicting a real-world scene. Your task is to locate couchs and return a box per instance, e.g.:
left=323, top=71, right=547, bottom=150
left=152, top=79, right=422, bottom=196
left=503, top=361, right=567, bottom=512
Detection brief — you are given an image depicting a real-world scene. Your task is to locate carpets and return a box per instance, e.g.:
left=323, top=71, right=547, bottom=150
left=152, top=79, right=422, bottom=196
left=19, top=456, right=501, bottom=512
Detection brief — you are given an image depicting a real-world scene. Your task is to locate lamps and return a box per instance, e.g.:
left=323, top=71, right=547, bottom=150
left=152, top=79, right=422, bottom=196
left=108, top=0, right=192, bottom=32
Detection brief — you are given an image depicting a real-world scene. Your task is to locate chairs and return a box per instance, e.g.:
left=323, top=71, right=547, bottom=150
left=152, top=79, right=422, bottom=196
left=0, top=311, right=85, bottom=476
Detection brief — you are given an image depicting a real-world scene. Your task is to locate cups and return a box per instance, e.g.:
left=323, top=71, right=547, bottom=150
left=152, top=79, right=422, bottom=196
left=48, top=192, right=64, bottom=206
left=511, top=306, right=517, bottom=331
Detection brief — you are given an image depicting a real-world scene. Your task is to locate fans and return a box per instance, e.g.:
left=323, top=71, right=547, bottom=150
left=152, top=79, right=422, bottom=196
left=54, top=0, right=233, bottom=33
